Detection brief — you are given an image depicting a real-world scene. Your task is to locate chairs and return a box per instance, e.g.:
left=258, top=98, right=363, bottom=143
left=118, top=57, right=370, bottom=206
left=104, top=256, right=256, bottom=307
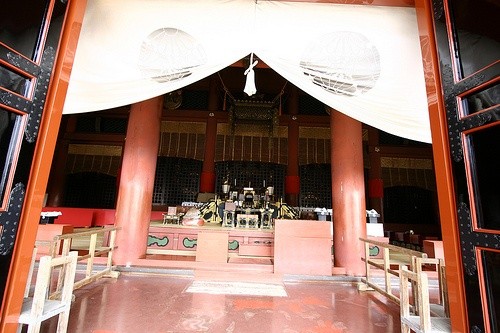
left=399, top=265, right=451, bottom=333
left=17, top=251, right=78, bottom=333
